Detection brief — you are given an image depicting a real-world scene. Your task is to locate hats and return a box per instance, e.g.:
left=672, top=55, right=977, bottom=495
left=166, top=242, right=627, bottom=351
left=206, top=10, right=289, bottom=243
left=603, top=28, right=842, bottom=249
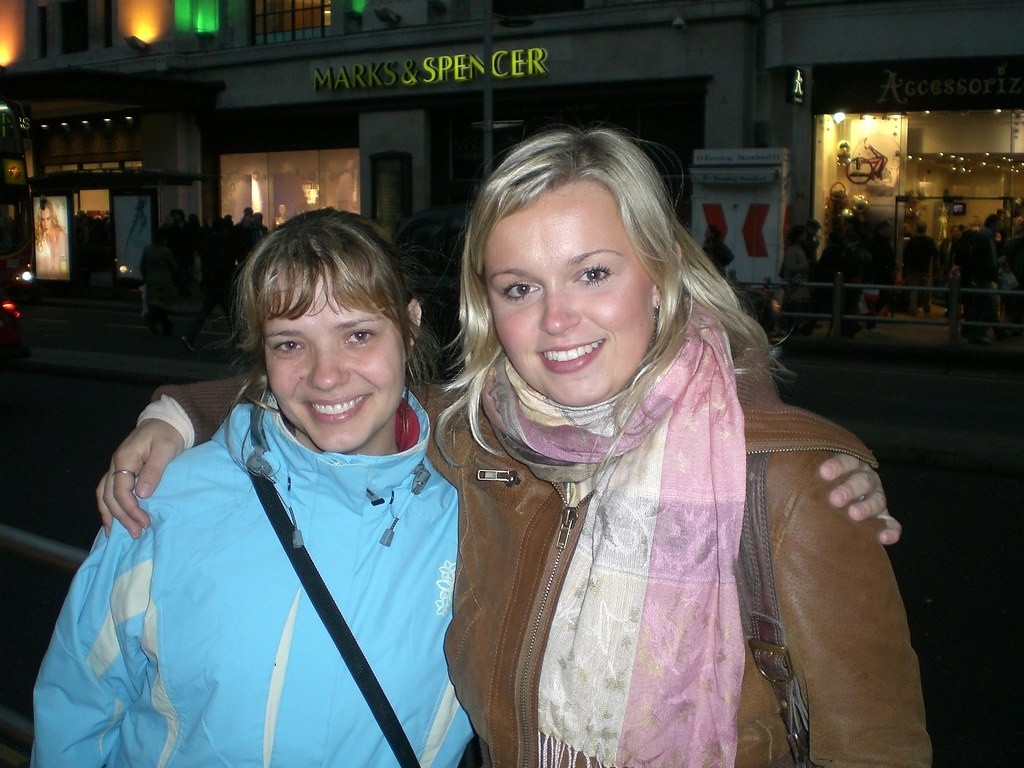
left=806, top=219, right=822, bottom=230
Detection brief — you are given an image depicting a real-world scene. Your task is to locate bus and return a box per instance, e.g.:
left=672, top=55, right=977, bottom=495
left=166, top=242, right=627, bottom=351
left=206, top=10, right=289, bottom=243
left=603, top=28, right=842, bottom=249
left=0, top=96, right=40, bottom=367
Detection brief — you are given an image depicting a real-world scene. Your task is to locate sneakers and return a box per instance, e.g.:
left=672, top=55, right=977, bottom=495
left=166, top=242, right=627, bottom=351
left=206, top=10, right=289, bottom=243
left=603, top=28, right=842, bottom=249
left=182, top=335, right=197, bottom=352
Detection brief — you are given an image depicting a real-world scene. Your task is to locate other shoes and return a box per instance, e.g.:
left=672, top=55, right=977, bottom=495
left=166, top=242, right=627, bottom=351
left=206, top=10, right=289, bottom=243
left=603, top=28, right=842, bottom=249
left=996, top=334, right=1007, bottom=341
left=163, top=322, right=176, bottom=334
left=925, top=311, right=930, bottom=315
left=800, top=328, right=811, bottom=336
left=144, top=321, right=161, bottom=335
left=911, top=310, right=917, bottom=316
left=788, top=326, right=799, bottom=336
left=977, top=338, right=992, bottom=347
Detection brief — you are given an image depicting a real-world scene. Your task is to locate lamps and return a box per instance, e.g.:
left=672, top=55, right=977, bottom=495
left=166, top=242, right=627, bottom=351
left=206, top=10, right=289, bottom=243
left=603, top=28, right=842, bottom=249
left=373, top=7, right=402, bottom=24
left=344, top=9, right=361, bottom=19
left=123, top=35, right=151, bottom=53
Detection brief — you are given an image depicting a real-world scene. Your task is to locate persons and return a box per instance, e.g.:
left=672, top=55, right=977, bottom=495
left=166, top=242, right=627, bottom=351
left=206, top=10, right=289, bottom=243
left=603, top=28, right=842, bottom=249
left=937, top=197, right=1024, bottom=346
left=28, top=129, right=936, bottom=768
left=35, top=200, right=67, bottom=274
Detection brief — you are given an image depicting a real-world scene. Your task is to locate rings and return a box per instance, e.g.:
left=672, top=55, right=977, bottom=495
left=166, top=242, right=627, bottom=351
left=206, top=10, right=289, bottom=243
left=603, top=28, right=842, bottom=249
left=113, top=470, right=135, bottom=479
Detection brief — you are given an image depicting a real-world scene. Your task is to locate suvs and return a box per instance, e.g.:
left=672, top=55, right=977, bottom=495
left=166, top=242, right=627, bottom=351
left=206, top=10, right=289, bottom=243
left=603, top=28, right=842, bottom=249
left=395, top=201, right=777, bottom=346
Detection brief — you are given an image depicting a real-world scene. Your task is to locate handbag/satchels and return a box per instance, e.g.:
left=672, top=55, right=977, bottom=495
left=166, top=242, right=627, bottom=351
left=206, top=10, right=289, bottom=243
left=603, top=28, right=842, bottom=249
left=953, top=263, right=987, bottom=300
left=138, top=284, right=148, bottom=317
